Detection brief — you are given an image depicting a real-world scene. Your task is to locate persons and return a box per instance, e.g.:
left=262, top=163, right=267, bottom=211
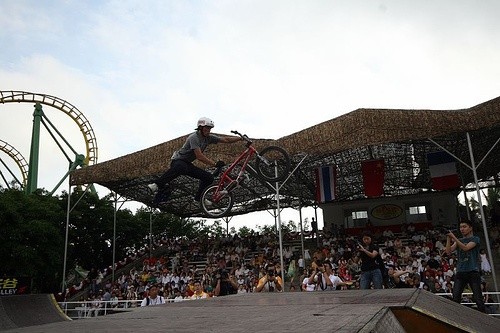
left=147, top=117, right=250, bottom=208
left=444, top=220, right=487, bottom=315
left=66, top=218, right=498, bottom=318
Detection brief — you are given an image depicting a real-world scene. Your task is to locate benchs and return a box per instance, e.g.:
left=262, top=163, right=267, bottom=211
left=167, top=239, right=302, bottom=272
left=353, top=232, right=410, bottom=250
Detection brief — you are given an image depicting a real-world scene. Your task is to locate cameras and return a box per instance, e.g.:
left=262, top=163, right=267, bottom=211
left=268, top=270, right=274, bottom=277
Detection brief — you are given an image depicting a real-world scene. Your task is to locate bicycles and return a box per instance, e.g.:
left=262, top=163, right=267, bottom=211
left=200, top=130, right=290, bottom=219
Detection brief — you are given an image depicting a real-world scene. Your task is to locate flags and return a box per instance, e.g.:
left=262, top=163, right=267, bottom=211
left=427, top=149, right=459, bottom=190
left=314, top=165, right=337, bottom=204
left=361, top=158, right=386, bottom=198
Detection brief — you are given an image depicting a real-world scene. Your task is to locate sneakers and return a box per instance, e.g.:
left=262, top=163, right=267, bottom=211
left=194, top=199, right=214, bottom=206
left=147, top=183, right=158, bottom=196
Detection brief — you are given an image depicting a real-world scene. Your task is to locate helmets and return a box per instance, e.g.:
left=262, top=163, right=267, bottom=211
left=197, top=117, right=214, bottom=127
